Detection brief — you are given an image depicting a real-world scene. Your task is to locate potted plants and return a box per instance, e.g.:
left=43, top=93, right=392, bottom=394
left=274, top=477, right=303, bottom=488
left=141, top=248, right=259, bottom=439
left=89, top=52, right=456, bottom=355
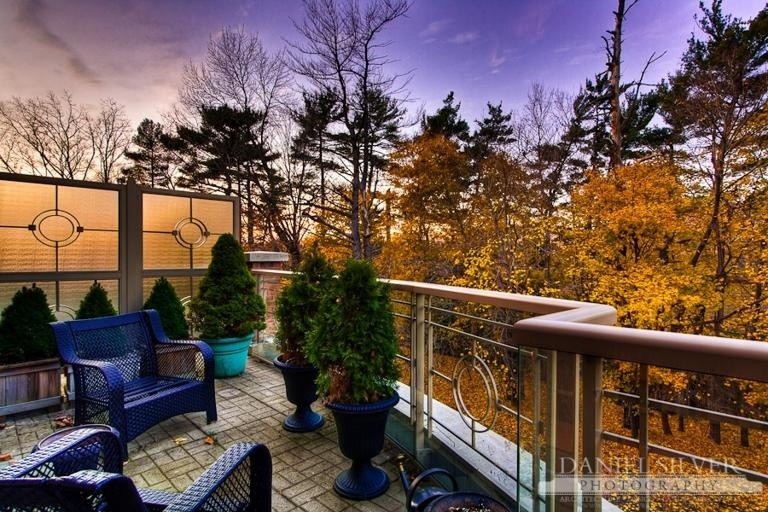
left=270, top=238, right=329, bottom=432
left=192, top=232, right=269, bottom=380
left=133, top=274, right=204, bottom=386
left=301, top=257, right=402, bottom=503
left=0, top=282, right=65, bottom=422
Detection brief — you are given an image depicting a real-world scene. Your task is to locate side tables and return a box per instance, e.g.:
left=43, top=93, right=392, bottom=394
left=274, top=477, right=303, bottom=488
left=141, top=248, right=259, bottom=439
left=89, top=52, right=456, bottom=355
left=24, top=421, right=124, bottom=478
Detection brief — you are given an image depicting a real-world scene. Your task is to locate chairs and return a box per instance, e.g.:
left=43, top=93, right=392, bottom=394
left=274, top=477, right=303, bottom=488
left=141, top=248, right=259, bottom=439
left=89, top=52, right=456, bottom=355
left=42, top=307, right=217, bottom=465
left=0, top=426, right=273, bottom=512
